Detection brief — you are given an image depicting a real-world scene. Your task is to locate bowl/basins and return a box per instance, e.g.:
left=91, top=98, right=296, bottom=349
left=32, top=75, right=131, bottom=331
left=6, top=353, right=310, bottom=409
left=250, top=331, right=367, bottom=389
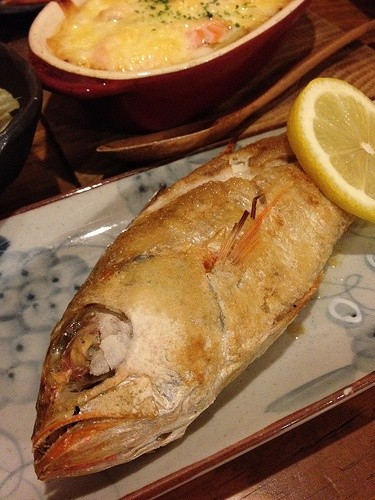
left=0, top=41, right=43, bottom=188
left=28, top=0, right=306, bottom=133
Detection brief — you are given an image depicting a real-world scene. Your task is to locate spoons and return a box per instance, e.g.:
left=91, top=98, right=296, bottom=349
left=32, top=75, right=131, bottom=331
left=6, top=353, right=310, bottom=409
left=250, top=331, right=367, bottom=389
left=95, top=20, right=375, bottom=159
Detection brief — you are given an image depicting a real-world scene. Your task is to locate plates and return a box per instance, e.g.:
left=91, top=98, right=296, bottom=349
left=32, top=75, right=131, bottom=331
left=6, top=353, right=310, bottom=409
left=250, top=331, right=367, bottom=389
left=0, top=99, right=375, bottom=500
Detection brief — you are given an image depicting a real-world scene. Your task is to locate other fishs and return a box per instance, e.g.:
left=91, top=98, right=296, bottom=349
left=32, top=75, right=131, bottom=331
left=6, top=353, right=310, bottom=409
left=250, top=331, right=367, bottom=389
left=31, top=131, right=356, bottom=482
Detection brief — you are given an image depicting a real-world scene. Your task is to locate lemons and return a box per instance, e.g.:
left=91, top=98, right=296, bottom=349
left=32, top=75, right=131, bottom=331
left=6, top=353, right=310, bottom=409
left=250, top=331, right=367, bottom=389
left=286, top=77, right=375, bottom=223
